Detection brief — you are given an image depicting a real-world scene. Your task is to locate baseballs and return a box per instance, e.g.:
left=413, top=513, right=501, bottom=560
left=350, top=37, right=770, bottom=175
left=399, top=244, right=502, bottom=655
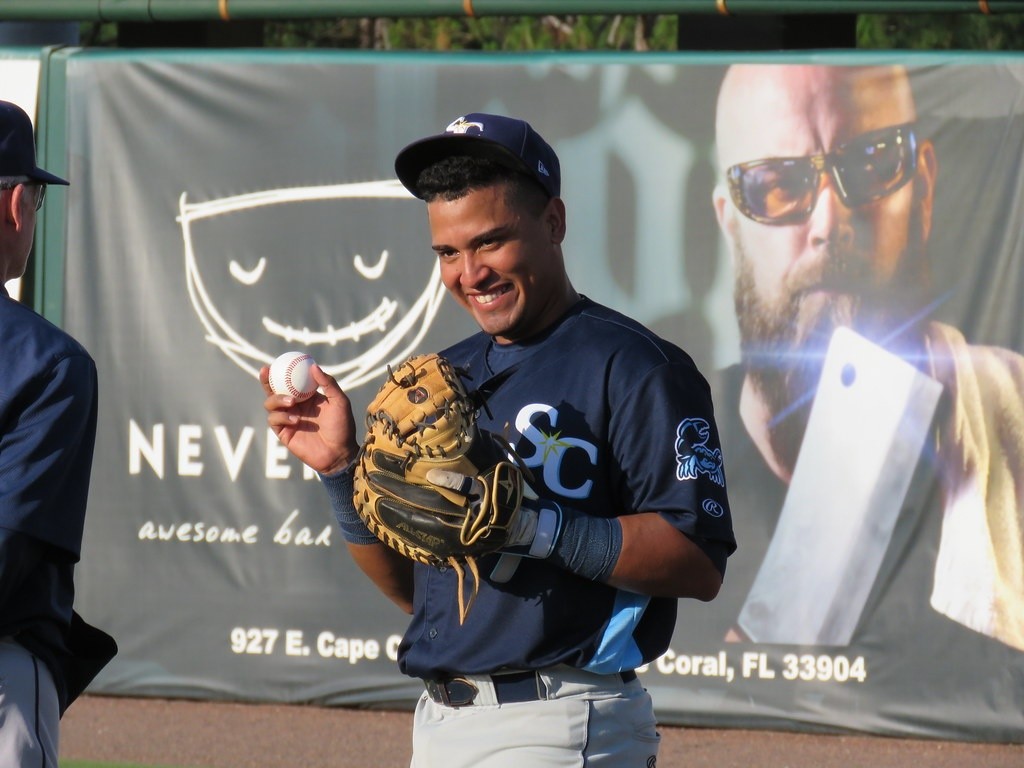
left=266, top=349, right=322, bottom=405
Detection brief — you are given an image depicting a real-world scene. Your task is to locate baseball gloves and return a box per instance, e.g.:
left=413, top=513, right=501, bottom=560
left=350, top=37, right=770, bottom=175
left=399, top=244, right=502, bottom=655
left=350, top=349, right=525, bottom=568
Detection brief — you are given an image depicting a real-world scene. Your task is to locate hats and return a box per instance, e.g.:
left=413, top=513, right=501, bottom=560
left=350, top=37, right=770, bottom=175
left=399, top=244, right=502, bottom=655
left=0, top=100, right=70, bottom=186
left=395, top=112, right=561, bottom=199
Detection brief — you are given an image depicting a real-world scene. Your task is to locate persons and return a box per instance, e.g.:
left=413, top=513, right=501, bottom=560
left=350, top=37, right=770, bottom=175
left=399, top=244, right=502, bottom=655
left=0, top=99, right=118, bottom=768
left=260, top=112, right=738, bottom=768
left=712, top=65, right=1024, bottom=645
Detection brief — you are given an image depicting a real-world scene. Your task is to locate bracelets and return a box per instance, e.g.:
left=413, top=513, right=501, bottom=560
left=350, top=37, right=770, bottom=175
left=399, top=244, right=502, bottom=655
left=317, top=464, right=381, bottom=545
left=551, top=517, right=623, bottom=582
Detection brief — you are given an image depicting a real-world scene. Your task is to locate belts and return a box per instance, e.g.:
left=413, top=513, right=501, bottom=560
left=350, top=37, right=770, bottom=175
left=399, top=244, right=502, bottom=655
left=423, top=670, right=637, bottom=707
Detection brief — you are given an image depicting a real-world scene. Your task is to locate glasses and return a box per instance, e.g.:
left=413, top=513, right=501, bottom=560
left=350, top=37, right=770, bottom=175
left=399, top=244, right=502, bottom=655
left=0, top=180, right=48, bottom=212
left=726, top=122, right=919, bottom=226
